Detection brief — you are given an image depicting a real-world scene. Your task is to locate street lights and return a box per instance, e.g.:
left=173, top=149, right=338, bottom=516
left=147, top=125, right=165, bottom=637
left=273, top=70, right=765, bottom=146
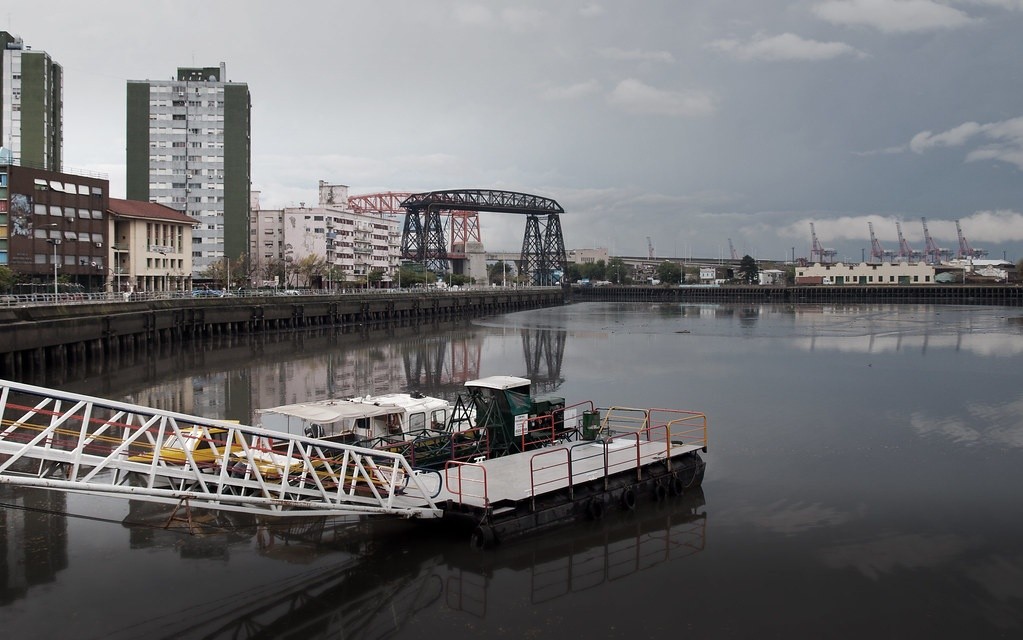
left=208, top=254, right=230, bottom=294
left=30, top=222, right=58, bottom=296
left=110, top=246, right=121, bottom=294
left=46, top=238, right=58, bottom=294
left=159, top=251, right=166, bottom=295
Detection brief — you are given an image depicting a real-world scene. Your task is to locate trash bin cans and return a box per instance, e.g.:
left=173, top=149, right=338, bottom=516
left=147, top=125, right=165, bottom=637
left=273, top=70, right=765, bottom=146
left=583, top=411, right=600, bottom=440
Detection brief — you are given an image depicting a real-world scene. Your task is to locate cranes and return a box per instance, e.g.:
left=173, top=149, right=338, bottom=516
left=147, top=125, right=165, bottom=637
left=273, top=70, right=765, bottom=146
left=642, top=212, right=978, bottom=262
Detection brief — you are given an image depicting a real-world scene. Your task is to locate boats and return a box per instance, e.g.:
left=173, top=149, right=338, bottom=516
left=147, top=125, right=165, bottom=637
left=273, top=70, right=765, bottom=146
left=125, top=374, right=567, bottom=474
